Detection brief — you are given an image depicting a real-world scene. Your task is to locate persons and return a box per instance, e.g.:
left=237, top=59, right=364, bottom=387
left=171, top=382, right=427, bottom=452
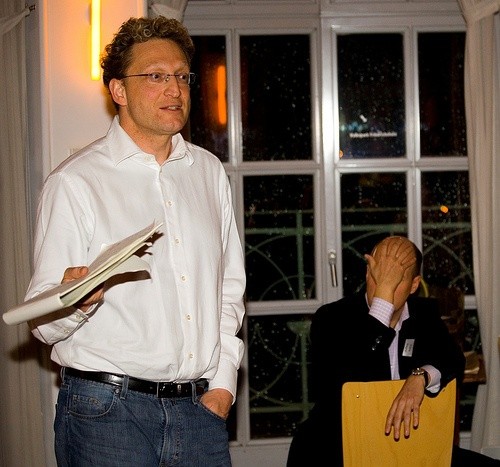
left=24, top=15, right=246, bottom=467
left=287, top=236, right=500, bottom=467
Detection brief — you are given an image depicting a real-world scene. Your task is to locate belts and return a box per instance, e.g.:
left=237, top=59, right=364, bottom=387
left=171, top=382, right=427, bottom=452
left=65, top=367, right=208, bottom=398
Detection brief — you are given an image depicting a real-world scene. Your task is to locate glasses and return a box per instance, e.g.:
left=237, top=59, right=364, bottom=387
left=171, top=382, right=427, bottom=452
left=108, top=73, right=196, bottom=95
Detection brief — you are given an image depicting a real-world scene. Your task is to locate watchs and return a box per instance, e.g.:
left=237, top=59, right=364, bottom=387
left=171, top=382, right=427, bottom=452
left=411, top=367, right=428, bottom=387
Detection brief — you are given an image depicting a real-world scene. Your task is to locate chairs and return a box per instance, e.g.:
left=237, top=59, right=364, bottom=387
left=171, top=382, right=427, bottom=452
left=341, top=377, right=456, bottom=467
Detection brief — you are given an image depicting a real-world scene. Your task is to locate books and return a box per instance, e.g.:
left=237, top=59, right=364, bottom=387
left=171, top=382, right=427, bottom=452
left=3, top=217, right=164, bottom=326
